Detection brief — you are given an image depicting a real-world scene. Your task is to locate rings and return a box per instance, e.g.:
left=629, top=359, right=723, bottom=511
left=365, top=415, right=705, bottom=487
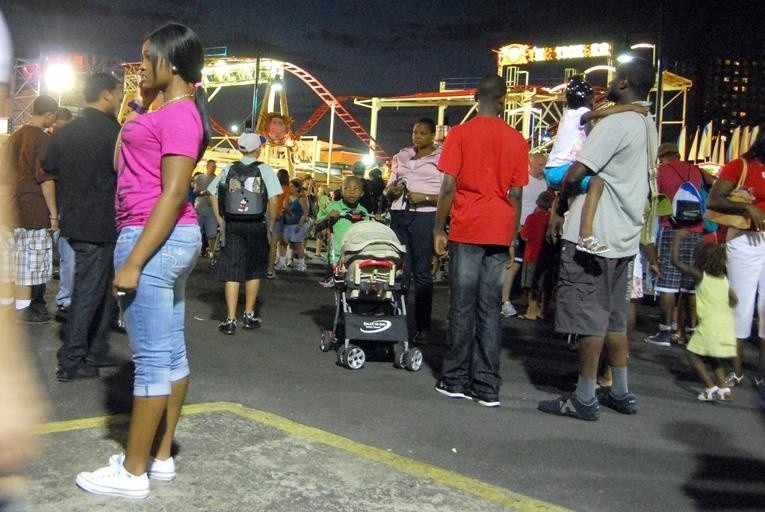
left=117, top=292, right=127, bottom=296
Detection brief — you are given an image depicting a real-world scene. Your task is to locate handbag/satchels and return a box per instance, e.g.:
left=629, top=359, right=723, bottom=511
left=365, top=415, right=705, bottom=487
left=704, top=180, right=756, bottom=230
left=386, top=179, right=416, bottom=227
left=640, top=193, right=659, bottom=245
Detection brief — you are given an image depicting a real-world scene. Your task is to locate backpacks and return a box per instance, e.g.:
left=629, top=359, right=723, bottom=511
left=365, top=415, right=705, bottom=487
left=223, top=161, right=267, bottom=221
left=666, top=161, right=703, bottom=228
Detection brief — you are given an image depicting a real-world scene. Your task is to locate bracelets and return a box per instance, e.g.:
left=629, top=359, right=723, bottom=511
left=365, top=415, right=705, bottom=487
left=49, top=216, right=59, bottom=221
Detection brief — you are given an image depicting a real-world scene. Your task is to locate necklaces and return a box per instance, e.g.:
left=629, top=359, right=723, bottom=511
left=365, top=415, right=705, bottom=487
left=151, top=94, right=195, bottom=111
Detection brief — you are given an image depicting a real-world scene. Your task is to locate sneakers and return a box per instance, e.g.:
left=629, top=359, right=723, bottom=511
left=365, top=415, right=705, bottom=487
left=604, top=387, right=639, bottom=414
left=644, top=324, right=696, bottom=346
left=1, top=296, right=69, bottom=323
left=500, top=300, right=544, bottom=320
left=75, top=465, right=150, bottom=500
left=219, top=320, right=237, bottom=335
left=537, top=391, right=600, bottom=420
left=243, top=316, right=262, bottom=329
left=109, top=454, right=176, bottom=481
left=57, top=356, right=118, bottom=381
left=267, top=247, right=335, bottom=288
left=434, top=381, right=501, bottom=408
left=412, top=331, right=427, bottom=344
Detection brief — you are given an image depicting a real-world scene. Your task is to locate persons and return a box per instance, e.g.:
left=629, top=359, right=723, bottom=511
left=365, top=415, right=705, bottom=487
left=191, top=161, right=389, bottom=287
left=40, top=72, right=122, bottom=381
left=384, top=118, right=443, bottom=346
left=52, top=107, right=76, bottom=323
left=0, top=96, right=60, bottom=325
left=544, top=76, right=650, bottom=255
left=574, top=125, right=765, bottom=404
left=433, top=75, right=530, bottom=407
left=501, top=152, right=559, bottom=322
left=207, top=132, right=284, bottom=335
left=74, top=23, right=213, bottom=500
left=545, top=59, right=659, bottom=422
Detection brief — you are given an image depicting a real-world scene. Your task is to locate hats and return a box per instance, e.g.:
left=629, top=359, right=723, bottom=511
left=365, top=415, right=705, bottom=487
left=352, top=161, right=365, bottom=171
left=657, top=143, right=679, bottom=156
left=566, top=74, right=593, bottom=108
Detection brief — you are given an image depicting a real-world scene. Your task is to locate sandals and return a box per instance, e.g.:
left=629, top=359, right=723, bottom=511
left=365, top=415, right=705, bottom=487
left=576, top=236, right=609, bottom=254
left=698, top=371, right=765, bottom=401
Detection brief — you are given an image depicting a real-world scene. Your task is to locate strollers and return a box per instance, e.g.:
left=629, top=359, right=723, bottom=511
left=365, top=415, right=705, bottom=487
left=319, top=211, right=423, bottom=372
left=207, top=231, right=219, bottom=269
